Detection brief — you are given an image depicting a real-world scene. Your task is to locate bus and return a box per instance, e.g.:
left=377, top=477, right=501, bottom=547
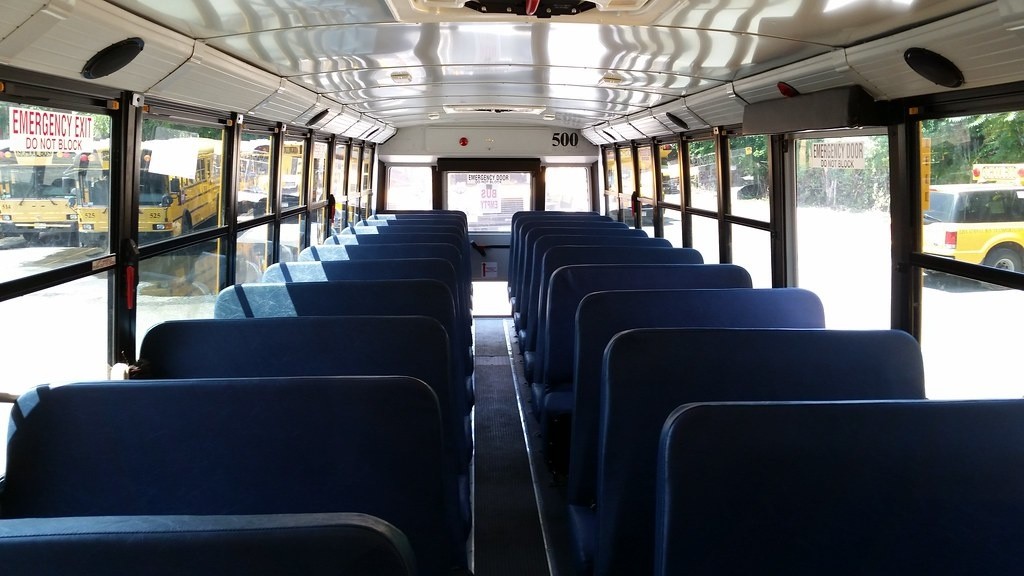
left=240, top=137, right=371, bottom=218
left=0, top=143, right=99, bottom=244
left=606, top=144, right=674, bottom=222
left=69, top=136, right=222, bottom=247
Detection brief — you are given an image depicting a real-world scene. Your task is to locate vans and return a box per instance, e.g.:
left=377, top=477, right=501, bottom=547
left=921, top=161, right=1024, bottom=291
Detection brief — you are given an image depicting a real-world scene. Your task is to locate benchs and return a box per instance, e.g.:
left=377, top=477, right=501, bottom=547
left=1, top=210, right=1023, bottom=576
